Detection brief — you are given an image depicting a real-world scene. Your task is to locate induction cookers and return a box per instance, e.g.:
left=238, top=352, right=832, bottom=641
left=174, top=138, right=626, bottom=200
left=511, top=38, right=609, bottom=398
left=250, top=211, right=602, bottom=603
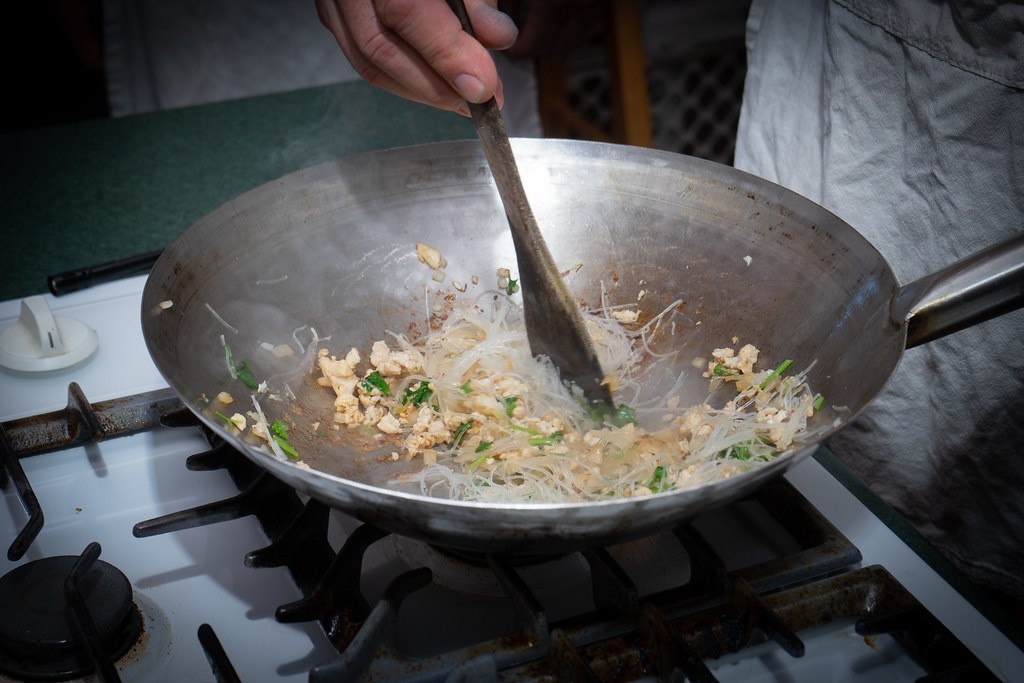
left=0, top=256, right=1024, bottom=683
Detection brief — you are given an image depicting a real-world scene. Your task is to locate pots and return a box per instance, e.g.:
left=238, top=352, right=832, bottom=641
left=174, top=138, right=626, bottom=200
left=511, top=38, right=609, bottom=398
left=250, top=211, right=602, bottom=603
left=139, top=137, right=1024, bottom=542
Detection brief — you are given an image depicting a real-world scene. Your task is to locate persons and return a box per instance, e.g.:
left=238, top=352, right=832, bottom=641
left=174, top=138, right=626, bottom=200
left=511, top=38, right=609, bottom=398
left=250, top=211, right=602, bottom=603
left=311, top=0, right=1022, bottom=602
